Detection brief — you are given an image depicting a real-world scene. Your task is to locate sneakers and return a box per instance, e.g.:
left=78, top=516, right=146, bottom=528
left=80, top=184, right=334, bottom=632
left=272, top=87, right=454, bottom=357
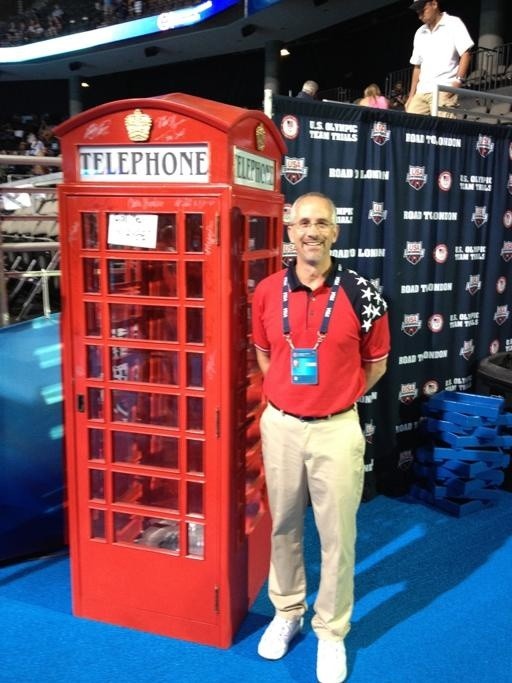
left=256, top=613, right=306, bottom=661
left=315, top=638, right=348, bottom=682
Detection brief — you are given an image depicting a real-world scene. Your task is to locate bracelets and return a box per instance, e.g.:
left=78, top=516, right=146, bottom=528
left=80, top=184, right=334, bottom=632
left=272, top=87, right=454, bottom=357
left=455, top=75, right=465, bottom=84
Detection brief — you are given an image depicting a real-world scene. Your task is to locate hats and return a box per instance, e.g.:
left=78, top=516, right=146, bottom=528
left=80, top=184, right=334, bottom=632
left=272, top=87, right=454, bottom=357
left=408, top=0, right=434, bottom=9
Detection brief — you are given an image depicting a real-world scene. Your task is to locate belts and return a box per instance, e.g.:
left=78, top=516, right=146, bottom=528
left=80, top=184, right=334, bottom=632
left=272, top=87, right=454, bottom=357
left=267, top=397, right=356, bottom=424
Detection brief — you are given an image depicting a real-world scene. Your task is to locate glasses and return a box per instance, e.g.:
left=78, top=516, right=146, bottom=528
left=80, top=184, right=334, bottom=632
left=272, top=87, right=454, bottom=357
left=289, top=220, right=337, bottom=231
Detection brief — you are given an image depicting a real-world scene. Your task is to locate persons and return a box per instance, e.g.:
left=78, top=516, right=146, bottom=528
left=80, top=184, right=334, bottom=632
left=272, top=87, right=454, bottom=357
left=1, top=0, right=199, bottom=48
left=249, top=190, right=394, bottom=682
left=400, top=0, right=477, bottom=119
left=2, top=107, right=76, bottom=183
left=296, top=79, right=319, bottom=101
left=386, top=79, right=409, bottom=109
left=360, top=82, right=388, bottom=111
